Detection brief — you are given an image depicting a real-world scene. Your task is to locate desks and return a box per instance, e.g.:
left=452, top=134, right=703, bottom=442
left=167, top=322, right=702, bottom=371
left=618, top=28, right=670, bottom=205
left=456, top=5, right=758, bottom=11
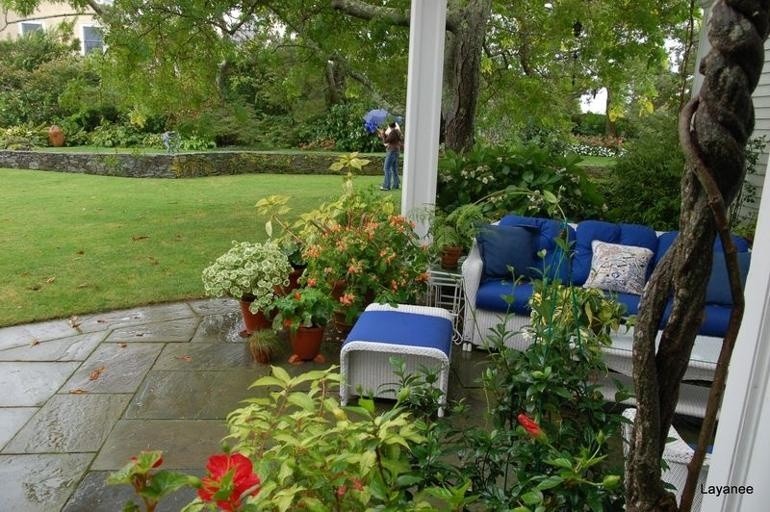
left=425, top=258, right=463, bottom=346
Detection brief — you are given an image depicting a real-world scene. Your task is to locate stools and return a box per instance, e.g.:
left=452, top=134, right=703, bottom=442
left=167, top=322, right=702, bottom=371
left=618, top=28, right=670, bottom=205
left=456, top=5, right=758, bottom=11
left=340, top=302, right=454, bottom=418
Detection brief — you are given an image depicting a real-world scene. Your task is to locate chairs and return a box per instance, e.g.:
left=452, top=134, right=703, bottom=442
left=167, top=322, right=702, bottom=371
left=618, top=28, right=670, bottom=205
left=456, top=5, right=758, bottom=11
left=622, top=408, right=712, bottom=512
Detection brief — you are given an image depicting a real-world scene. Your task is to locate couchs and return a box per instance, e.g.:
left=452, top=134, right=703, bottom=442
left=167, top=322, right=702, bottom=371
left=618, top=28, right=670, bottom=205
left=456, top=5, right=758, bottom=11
left=461, top=221, right=752, bottom=382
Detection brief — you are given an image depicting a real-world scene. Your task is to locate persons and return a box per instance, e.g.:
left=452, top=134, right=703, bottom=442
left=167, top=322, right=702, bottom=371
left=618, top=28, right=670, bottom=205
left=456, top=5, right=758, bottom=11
left=376, top=121, right=402, bottom=151
left=378, top=120, right=400, bottom=191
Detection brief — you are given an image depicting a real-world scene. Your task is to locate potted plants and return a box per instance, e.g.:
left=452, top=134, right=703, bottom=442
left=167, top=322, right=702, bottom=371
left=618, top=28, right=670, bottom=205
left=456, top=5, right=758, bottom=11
left=200, top=236, right=295, bottom=335
left=46, top=115, right=66, bottom=146
left=407, top=203, right=492, bottom=268
left=249, top=328, right=285, bottom=363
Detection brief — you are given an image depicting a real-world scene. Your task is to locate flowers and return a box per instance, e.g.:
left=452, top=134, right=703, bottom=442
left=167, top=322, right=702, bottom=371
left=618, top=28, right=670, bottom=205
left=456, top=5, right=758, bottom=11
left=253, top=151, right=432, bottom=335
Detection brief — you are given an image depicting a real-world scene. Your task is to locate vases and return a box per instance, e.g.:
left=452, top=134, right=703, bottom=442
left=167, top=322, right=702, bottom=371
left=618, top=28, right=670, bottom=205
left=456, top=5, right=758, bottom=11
left=266, top=265, right=377, bottom=360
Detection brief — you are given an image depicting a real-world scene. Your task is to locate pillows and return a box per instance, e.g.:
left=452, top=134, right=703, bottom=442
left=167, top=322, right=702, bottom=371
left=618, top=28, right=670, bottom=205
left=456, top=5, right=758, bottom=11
left=475, top=216, right=751, bottom=308
